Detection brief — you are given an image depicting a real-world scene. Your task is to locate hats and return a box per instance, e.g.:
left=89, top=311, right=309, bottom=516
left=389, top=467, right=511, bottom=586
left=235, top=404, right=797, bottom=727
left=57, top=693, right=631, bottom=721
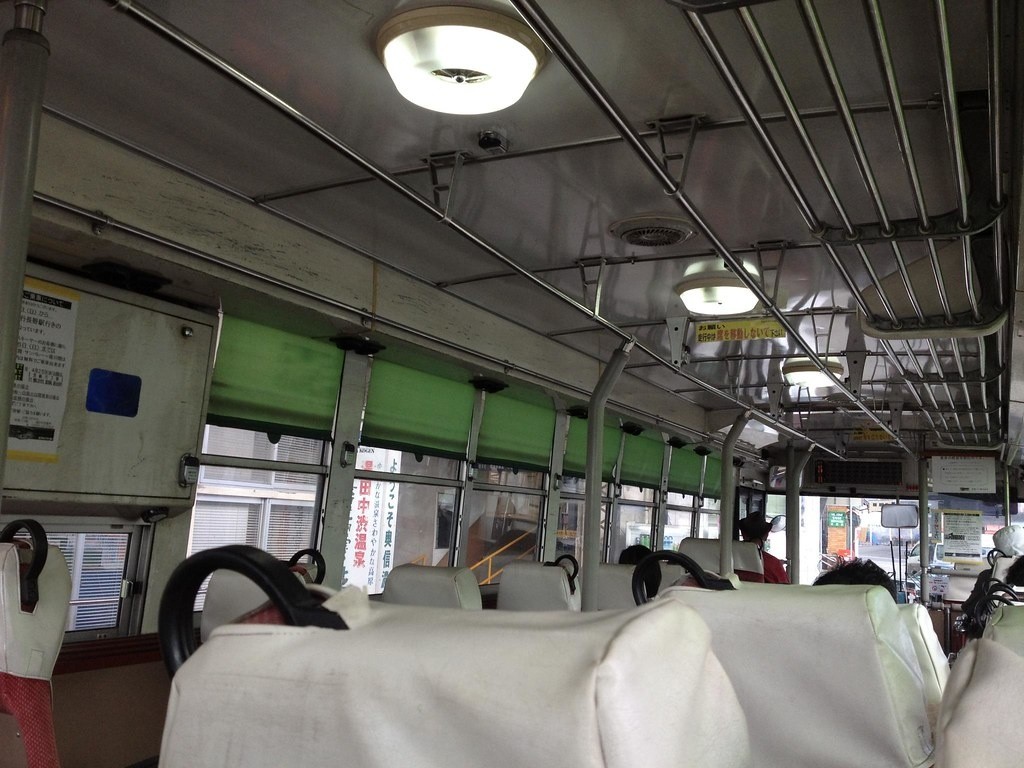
left=736, top=510, right=774, bottom=535
left=993, top=525, right=1024, bottom=556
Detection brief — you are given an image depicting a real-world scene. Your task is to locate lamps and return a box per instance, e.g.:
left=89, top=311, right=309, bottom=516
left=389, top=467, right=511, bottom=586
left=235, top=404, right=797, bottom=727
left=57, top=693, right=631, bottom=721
left=677, top=280, right=762, bottom=316
left=781, top=356, right=845, bottom=369
left=375, top=5, right=545, bottom=116
left=674, top=261, right=761, bottom=288
left=783, top=366, right=845, bottom=388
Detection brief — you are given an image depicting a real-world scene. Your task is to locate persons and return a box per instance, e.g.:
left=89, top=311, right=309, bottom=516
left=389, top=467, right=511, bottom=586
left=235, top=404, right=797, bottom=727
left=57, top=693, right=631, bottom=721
left=814, top=559, right=897, bottom=603
left=619, top=545, right=662, bottom=598
left=736, top=509, right=790, bottom=584
left=960, top=525, right=1024, bottom=639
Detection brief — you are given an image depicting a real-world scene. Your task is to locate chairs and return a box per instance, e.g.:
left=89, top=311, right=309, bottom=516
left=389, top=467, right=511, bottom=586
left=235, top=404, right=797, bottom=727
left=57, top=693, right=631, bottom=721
left=0, top=519, right=73, bottom=768
left=154, top=538, right=1024, bottom=768
